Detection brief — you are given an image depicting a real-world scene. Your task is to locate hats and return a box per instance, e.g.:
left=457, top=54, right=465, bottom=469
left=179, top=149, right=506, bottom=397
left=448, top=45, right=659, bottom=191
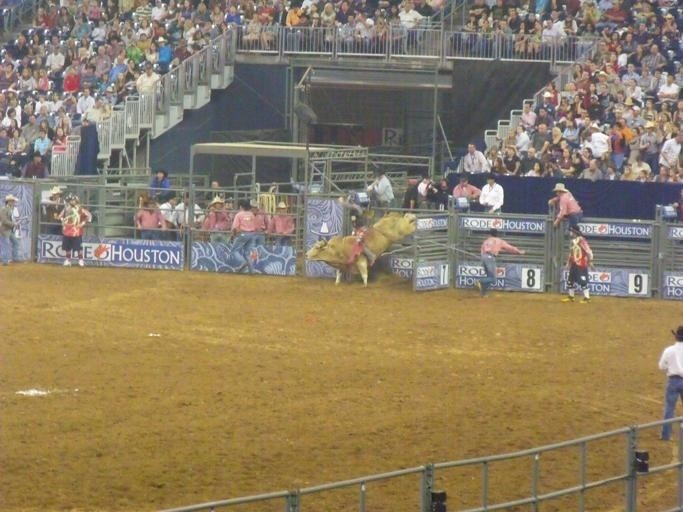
left=643, top=121, right=657, bottom=128
left=631, top=105, right=641, bottom=112
left=155, top=36, right=168, bottom=44
left=550, top=182, right=568, bottom=194
left=275, top=201, right=289, bottom=211
left=3, top=194, right=19, bottom=202
left=206, top=195, right=224, bottom=208
left=64, top=192, right=80, bottom=200
left=670, top=325, right=683, bottom=339
left=48, top=186, right=63, bottom=198
left=566, top=224, right=583, bottom=237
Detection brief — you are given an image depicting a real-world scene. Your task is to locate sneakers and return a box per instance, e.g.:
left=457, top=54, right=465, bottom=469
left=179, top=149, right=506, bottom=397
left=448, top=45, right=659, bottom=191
left=78, top=259, right=85, bottom=267
left=474, top=280, right=481, bottom=289
left=578, top=296, right=592, bottom=305
left=561, top=295, right=576, bottom=303
left=63, top=259, right=71, bottom=266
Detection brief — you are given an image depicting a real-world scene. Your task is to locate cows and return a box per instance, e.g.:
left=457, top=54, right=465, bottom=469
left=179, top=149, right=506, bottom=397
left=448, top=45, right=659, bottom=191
left=304, top=210, right=417, bottom=289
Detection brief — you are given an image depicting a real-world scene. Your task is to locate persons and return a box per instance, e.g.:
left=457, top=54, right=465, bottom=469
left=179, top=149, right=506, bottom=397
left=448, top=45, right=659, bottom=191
left=558, top=223, right=596, bottom=304
left=473, top=225, right=527, bottom=295
left=226, top=199, right=262, bottom=274
left=58, top=193, right=94, bottom=268
left=0, top=193, right=23, bottom=267
left=1, top=1, right=682, bottom=241
left=10, top=199, right=23, bottom=263
left=655, top=324, right=683, bottom=443
left=336, top=192, right=376, bottom=267
